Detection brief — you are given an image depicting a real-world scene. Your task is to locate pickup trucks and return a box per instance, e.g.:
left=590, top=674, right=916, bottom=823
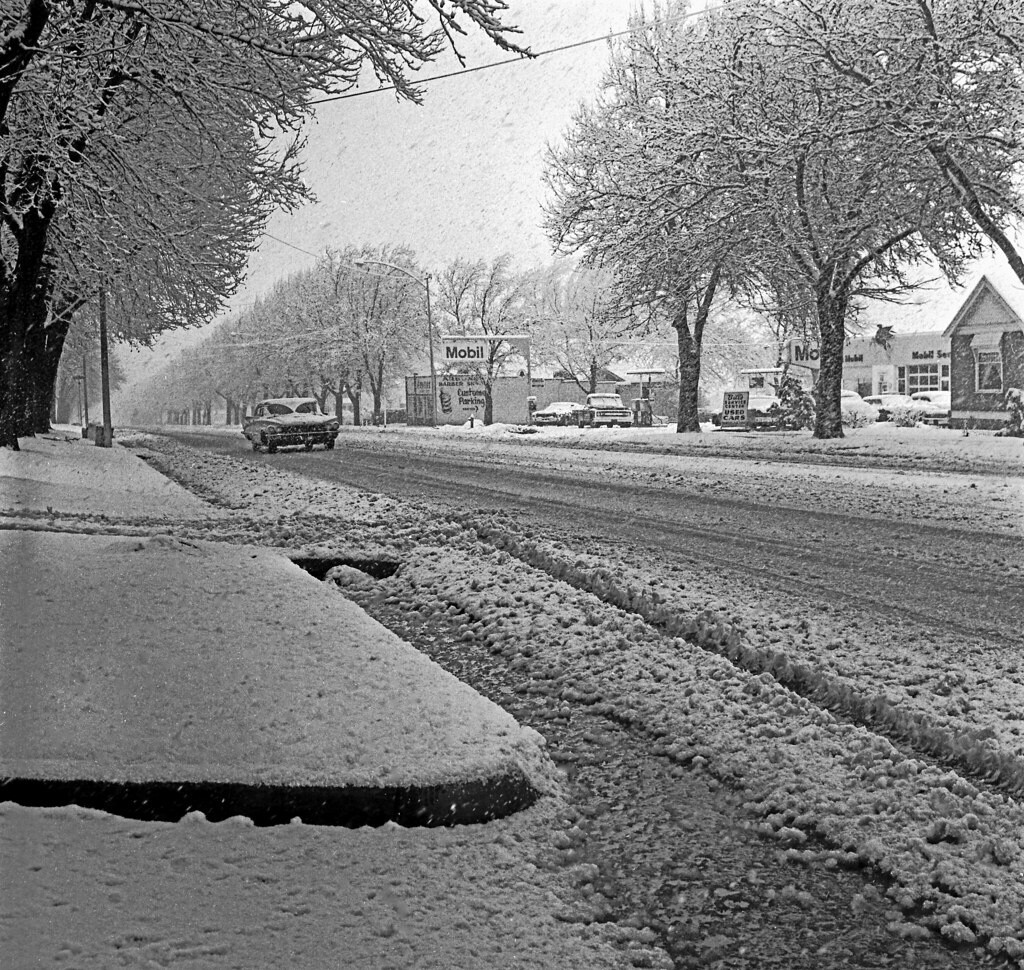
left=586, top=393, right=632, bottom=428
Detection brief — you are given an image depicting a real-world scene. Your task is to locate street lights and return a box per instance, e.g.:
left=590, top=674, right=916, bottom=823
left=354, top=259, right=438, bottom=428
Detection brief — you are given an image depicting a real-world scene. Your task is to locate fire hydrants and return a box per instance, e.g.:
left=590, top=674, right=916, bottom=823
left=468, top=415, right=474, bottom=428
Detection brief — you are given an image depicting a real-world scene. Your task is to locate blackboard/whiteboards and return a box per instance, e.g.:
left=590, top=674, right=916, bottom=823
left=721, top=391, right=750, bottom=424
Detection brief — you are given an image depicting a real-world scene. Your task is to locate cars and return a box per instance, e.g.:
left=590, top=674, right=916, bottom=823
left=840, top=389, right=951, bottom=425
left=239, top=397, right=340, bottom=454
left=530, top=402, right=584, bottom=427
